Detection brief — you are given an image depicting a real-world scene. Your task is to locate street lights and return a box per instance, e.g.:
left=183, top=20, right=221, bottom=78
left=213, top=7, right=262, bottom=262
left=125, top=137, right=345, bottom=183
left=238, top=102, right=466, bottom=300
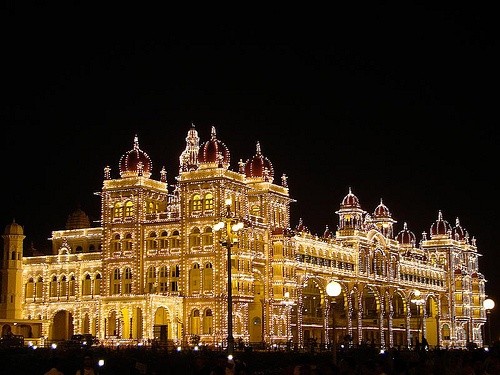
left=326, top=280, right=341, bottom=359
left=213, top=199, right=243, bottom=352
left=411, top=289, right=425, bottom=350
left=483, top=298, right=494, bottom=356
